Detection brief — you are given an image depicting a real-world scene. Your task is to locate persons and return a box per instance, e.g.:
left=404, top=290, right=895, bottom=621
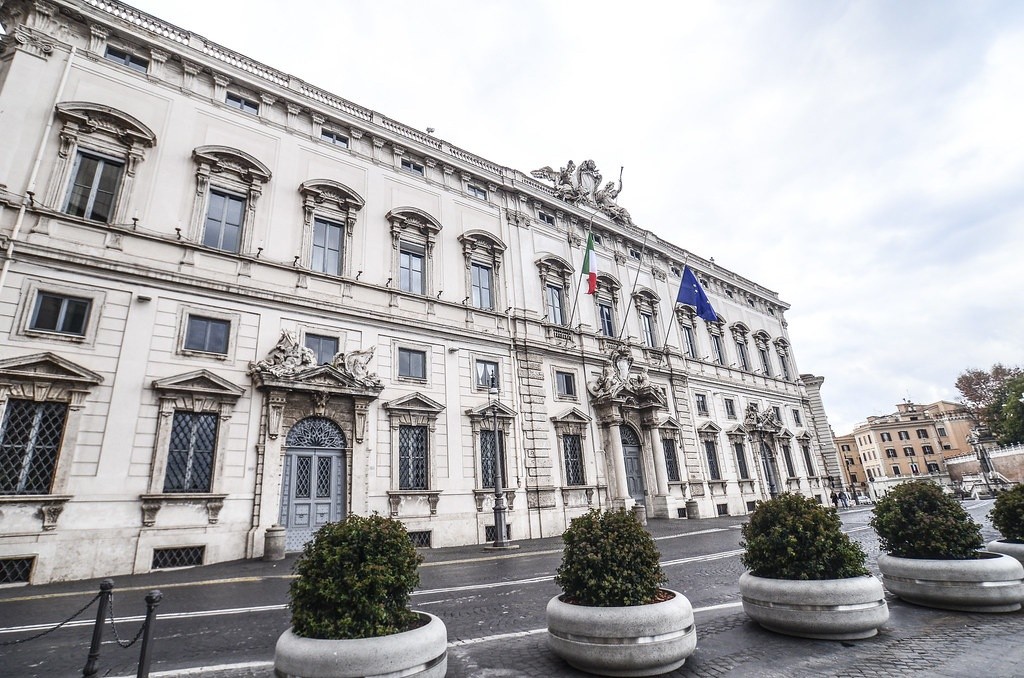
left=830, top=490, right=839, bottom=509
left=553, top=164, right=635, bottom=228
left=838, top=491, right=849, bottom=509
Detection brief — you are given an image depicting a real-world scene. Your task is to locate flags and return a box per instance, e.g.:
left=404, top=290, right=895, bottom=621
left=581, top=230, right=598, bottom=295
left=676, top=263, right=720, bottom=322
